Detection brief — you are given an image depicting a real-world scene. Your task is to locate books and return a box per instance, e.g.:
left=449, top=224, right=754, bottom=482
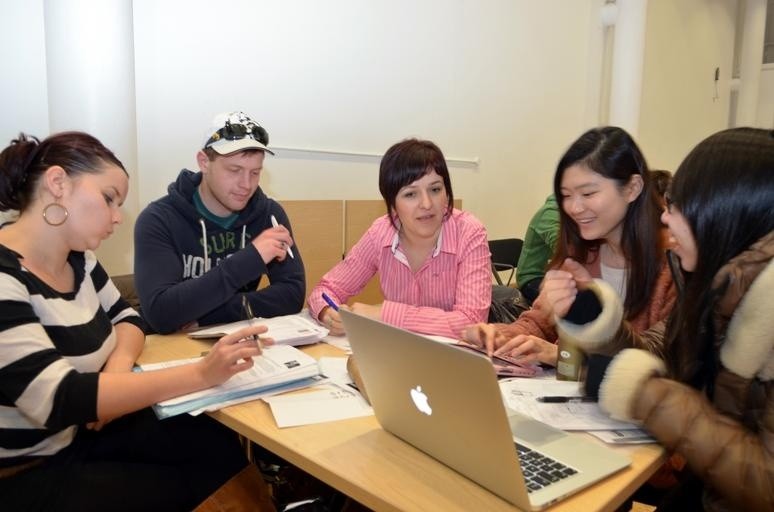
left=124, top=343, right=322, bottom=424
left=446, top=341, right=545, bottom=379
left=187, top=314, right=329, bottom=348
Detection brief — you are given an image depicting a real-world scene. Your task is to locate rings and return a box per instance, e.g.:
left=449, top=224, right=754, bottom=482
left=279, top=242, right=286, bottom=250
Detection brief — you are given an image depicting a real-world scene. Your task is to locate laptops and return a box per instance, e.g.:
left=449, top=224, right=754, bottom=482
left=338, top=305, right=632, bottom=512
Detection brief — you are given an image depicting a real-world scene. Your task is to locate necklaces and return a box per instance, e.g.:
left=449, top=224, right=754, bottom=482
left=512, top=190, right=565, bottom=306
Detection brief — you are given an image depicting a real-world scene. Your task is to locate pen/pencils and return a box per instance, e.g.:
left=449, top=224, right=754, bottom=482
left=537, top=396, right=596, bottom=402
left=242, top=296, right=264, bottom=356
left=321, top=292, right=340, bottom=316
left=270, top=215, right=295, bottom=263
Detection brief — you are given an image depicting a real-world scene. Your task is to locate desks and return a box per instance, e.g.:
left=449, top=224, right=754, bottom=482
left=134, top=307, right=672, bottom=512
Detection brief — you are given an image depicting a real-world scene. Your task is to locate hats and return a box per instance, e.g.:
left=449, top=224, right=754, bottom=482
left=194, top=110, right=276, bottom=156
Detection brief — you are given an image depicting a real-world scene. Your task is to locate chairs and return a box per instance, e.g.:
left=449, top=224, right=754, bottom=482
left=488, top=239, right=523, bottom=290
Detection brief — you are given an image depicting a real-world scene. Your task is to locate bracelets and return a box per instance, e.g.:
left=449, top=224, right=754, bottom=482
left=318, top=305, right=332, bottom=322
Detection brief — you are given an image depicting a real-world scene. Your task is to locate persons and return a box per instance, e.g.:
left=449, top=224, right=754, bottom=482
left=647, top=167, right=671, bottom=215
left=458, top=123, right=686, bottom=370
left=130, top=108, right=305, bottom=336
left=307, top=137, right=490, bottom=339
left=0, top=128, right=273, bottom=512
left=541, top=124, right=772, bottom=512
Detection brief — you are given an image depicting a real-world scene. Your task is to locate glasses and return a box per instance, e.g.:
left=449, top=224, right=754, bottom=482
left=221, top=124, right=268, bottom=147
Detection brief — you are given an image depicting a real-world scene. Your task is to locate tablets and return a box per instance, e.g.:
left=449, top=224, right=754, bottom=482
left=453, top=344, right=538, bottom=378
